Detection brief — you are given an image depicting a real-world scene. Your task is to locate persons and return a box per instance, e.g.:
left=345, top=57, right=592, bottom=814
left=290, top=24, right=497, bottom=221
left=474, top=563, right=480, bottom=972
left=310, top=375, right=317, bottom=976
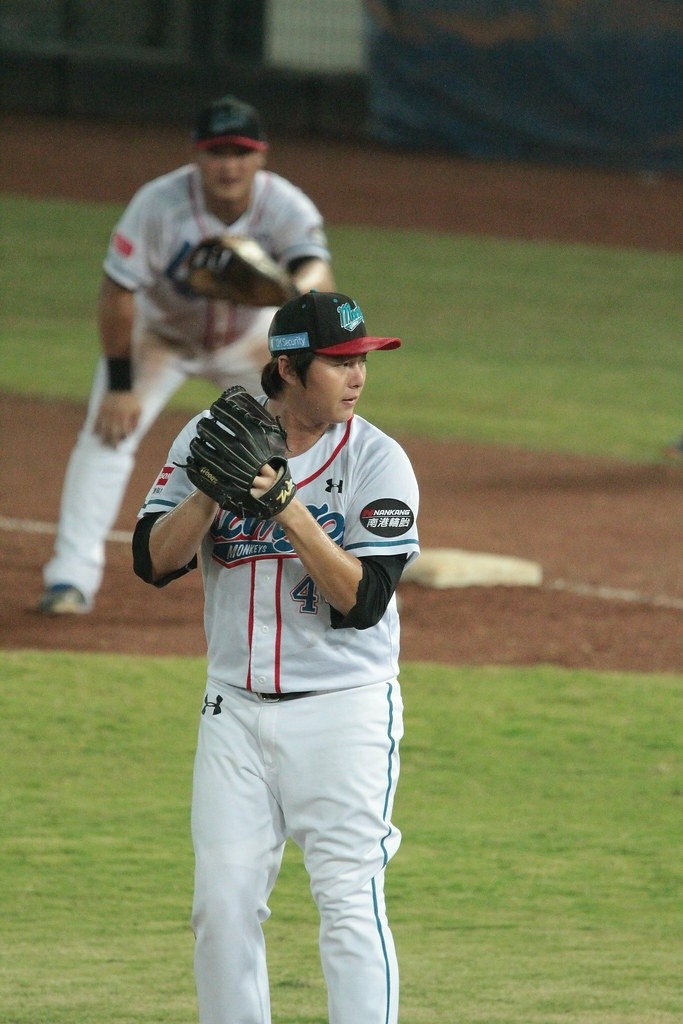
left=41, top=98, right=335, bottom=616
left=131, top=288, right=420, bottom=1024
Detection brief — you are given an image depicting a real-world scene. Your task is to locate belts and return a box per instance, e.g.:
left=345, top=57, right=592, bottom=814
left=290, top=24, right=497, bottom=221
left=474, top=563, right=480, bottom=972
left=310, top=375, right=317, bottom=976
left=251, top=688, right=316, bottom=703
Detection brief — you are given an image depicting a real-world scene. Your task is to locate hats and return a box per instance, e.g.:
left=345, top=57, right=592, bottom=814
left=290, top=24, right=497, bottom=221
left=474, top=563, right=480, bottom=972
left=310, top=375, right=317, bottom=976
left=267, top=286, right=404, bottom=356
left=193, top=97, right=267, bottom=152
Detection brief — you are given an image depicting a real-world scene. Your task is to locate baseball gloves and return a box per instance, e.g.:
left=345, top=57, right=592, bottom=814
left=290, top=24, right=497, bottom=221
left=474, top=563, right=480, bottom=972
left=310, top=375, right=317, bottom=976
left=169, top=236, right=301, bottom=308
left=170, top=384, right=298, bottom=523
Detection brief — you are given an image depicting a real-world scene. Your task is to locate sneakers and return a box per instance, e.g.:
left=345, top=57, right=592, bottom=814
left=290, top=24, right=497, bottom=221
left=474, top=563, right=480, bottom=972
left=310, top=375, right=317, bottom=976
left=41, top=586, right=91, bottom=614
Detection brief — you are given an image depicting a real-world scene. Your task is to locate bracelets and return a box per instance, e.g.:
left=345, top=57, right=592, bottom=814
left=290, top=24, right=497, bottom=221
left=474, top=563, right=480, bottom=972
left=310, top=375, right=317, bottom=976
left=103, top=356, right=134, bottom=390
